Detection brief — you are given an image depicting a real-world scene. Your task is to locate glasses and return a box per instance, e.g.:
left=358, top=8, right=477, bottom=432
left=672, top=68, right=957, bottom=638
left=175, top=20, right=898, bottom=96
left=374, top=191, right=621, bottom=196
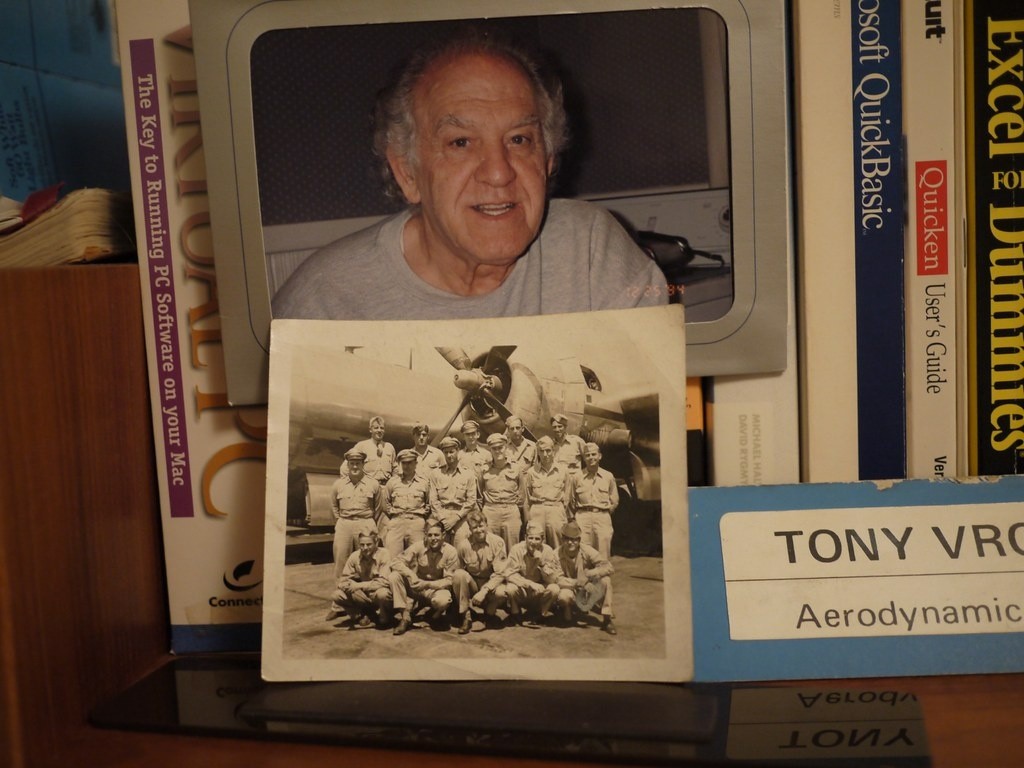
left=633, top=228, right=725, bottom=285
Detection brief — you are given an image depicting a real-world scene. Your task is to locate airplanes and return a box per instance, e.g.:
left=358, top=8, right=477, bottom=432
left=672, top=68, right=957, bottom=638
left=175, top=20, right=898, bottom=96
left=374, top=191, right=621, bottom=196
left=287, top=335, right=660, bottom=554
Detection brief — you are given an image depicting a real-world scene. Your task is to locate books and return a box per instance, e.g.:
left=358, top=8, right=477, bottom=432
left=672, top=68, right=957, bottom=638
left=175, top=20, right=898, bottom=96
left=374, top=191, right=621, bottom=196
left=0, top=189, right=134, bottom=267
left=115, top=0, right=1024, bottom=660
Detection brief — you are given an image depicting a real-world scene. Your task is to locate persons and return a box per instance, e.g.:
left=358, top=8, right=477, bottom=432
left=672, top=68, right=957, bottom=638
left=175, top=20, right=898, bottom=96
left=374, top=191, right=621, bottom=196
left=328, top=413, right=618, bottom=636
left=271, top=29, right=671, bottom=318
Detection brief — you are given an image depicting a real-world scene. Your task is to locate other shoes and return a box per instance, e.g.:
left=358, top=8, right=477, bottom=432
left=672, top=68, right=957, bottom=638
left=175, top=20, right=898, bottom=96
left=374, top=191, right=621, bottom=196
left=326, top=609, right=617, bottom=635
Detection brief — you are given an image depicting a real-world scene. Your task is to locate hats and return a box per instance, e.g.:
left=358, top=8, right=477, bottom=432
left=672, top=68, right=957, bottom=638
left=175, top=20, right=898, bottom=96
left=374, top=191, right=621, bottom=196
left=358, top=527, right=378, bottom=538
left=537, top=435, right=553, bottom=448
left=584, top=442, right=600, bottom=453
left=549, top=414, right=567, bottom=426
left=560, top=521, right=582, bottom=539
left=505, top=415, right=521, bottom=425
left=397, top=449, right=419, bottom=462
left=460, top=420, right=478, bottom=434
left=526, top=520, right=545, bottom=536
left=424, top=519, right=445, bottom=526
left=343, top=450, right=366, bottom=461
left=412, top=422, right=428, bottom=435
left=486, top=433, right=508, bottom=447
left=439, top=438, right=461, bottom=450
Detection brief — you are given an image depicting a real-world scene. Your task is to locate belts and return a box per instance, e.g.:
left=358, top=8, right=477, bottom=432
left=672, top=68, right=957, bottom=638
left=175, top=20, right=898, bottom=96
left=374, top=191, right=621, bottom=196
left=581, top=507, right=607, bottom=512
left=534, top=501, right=559, bottom=505
left=484, top=502, right=514, bottom=508
left=396, top=513, right=423, bottom=517
left=442, top=505, right=462, bottom=510
left=378, top=480, right=386, bottom=483
left=347, top=514, right=365, bottom=520
